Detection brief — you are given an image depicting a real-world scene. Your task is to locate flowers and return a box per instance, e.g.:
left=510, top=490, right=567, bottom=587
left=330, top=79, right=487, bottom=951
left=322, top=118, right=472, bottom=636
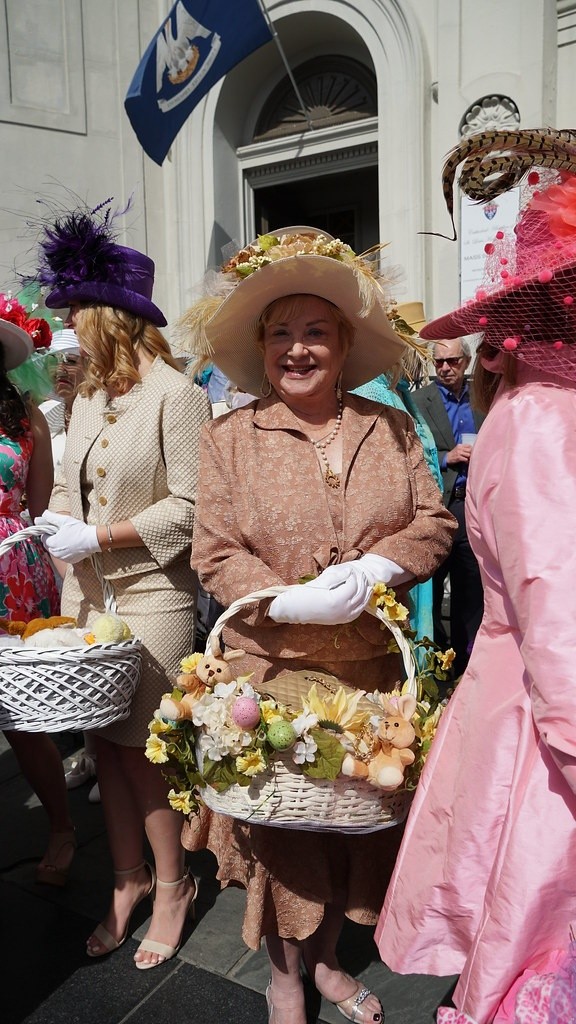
left=221, top=227, right=358, bottom=278
left=144, top=586, right=460, bottom=814
left=0, top=291, right=53, bottom=349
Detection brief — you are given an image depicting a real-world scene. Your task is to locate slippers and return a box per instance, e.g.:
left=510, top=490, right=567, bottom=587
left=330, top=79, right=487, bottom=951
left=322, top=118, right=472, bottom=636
left=299, top=951, right=384, bottom=1024
left=35, top=824, right=78, bottom=882
left=266, top=978, right=307, bottom=1024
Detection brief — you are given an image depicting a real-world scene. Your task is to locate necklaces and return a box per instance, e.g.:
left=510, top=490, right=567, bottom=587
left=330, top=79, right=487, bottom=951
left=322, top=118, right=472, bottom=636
left=308, top=389, right=343, bottom=489
left=64, top=410, right=71, bottom=433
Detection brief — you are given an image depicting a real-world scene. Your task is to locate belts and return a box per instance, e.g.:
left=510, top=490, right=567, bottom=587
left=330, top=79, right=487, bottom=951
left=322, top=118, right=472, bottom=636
left=452, top=485, right=468, bottom=498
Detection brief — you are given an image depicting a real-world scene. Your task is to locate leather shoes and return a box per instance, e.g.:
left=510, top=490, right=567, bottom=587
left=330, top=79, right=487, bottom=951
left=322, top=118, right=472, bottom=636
left=65, top=751, right=99, bottom=790
left=88, top=782, right=102, bottom=803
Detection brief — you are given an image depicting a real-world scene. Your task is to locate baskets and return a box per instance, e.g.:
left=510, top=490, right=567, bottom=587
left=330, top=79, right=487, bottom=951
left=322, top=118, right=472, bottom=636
left=1, top=525, right=144, bottom=733
left=197, top=585, right=417, bottom=833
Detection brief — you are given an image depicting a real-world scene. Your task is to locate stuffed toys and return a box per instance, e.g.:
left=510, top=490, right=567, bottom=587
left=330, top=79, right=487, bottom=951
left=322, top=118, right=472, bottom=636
left=342, top=693, right=416, bottom=791
left=160, top=635, right=245, bottom=721
left=9, top=614, right=131, bottom=649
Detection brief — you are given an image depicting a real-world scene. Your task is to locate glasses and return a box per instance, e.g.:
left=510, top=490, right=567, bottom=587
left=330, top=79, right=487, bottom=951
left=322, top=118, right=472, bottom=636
left=431, top=354, right=469, bottom=367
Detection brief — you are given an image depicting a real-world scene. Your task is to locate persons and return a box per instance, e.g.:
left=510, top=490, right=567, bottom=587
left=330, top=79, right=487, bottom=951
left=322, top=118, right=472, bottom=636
left=36, top=329, right=101, bottom=802
left=371, top=170, right=575, bottom=1023
left=348, top=302, right=483, bottom=685
left=33, top=213, right=213, bottom=969
left=0, top=292, right=77, bottom=888
left=171, top=348, right=258, bottom=409
left=190, top=226, right=459, bottom=1024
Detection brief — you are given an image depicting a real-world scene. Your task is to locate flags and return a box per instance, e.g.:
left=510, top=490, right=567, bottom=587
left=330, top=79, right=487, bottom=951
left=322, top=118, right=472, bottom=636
left=123, top=0, right=273, bottom=167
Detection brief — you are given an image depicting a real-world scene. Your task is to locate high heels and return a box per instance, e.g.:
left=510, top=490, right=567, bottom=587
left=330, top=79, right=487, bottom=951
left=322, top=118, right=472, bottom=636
left=133, top=865, right=200, bottom=969
left=86, top=859, right=156, bottom=956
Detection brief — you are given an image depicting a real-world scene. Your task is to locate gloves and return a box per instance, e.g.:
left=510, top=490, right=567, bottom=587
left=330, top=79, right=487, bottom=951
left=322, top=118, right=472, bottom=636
left=34, top=509, right=103, bottom=564
left=306, top=561, right=373, bottom=606
left=265, top=566, right=374, bottom=624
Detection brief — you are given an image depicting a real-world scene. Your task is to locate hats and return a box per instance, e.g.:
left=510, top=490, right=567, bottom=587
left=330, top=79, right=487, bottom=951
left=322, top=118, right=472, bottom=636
left=4, top=175, right=168, bottom=327
left=421, top=129, right=576, bottom=381
left=167, top=224, right=449, bottom=402
left=389, top=301, right=427, bottom=344
left=6, top=284, right=86, bottom=404
left=0, top=293, right=52, bottom=371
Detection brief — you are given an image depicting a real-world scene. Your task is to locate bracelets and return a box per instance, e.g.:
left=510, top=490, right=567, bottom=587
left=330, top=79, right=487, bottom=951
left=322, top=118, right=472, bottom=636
left=106, top=524, right=113, bottom=553
